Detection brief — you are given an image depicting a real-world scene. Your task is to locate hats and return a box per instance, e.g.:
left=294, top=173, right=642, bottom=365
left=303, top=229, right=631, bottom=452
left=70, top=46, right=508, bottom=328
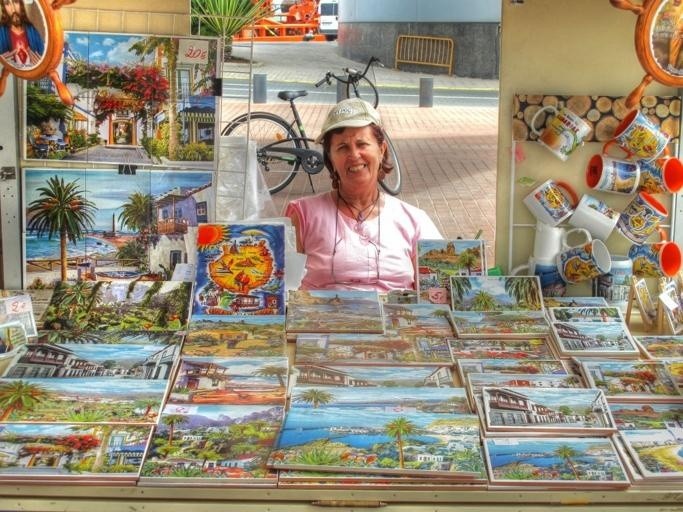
left=315, top=97, right=383, bottom=145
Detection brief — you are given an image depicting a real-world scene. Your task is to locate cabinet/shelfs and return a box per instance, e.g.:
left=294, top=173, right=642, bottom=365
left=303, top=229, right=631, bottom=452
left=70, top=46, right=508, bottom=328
left=510, top=96, right=681, bottom=314
left=16, top=6, right=254, bottom=288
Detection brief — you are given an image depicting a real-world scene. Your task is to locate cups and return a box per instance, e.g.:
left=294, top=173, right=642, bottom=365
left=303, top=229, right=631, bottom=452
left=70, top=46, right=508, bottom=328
left=615, top=191, right=668, bottom=247
left=521, top=178, right=578, bottom=226
left=628, top=229, right=681, bottom=278
left=636, top=156, right=683, bottom=194
left=566, top=194, right=621, bottom=242
left=530, top=106, right=592, bottom=162
left=585, top=140, right=640, bottom=195
left=555, top=228, right=611, bottom=286
left=602, top=109, right=671, bottom=163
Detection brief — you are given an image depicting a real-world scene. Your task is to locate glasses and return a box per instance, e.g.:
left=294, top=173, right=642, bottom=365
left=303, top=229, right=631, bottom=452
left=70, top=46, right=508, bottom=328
left=331, top=237, right=380, bottom=286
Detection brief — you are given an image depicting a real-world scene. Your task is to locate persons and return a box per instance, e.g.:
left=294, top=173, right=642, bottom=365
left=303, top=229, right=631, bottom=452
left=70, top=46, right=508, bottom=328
left=1, top=0, right=44, bottom=66
left=285, top=98, right=444, bottom=296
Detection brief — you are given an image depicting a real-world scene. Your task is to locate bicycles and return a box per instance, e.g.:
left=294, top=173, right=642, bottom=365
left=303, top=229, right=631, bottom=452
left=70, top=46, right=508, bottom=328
left=221, top=55, right=402, bottom=194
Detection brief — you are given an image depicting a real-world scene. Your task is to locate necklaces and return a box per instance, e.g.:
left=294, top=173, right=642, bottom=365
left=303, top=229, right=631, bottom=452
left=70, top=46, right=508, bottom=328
left=337, top=190, right=380, bottom=232
